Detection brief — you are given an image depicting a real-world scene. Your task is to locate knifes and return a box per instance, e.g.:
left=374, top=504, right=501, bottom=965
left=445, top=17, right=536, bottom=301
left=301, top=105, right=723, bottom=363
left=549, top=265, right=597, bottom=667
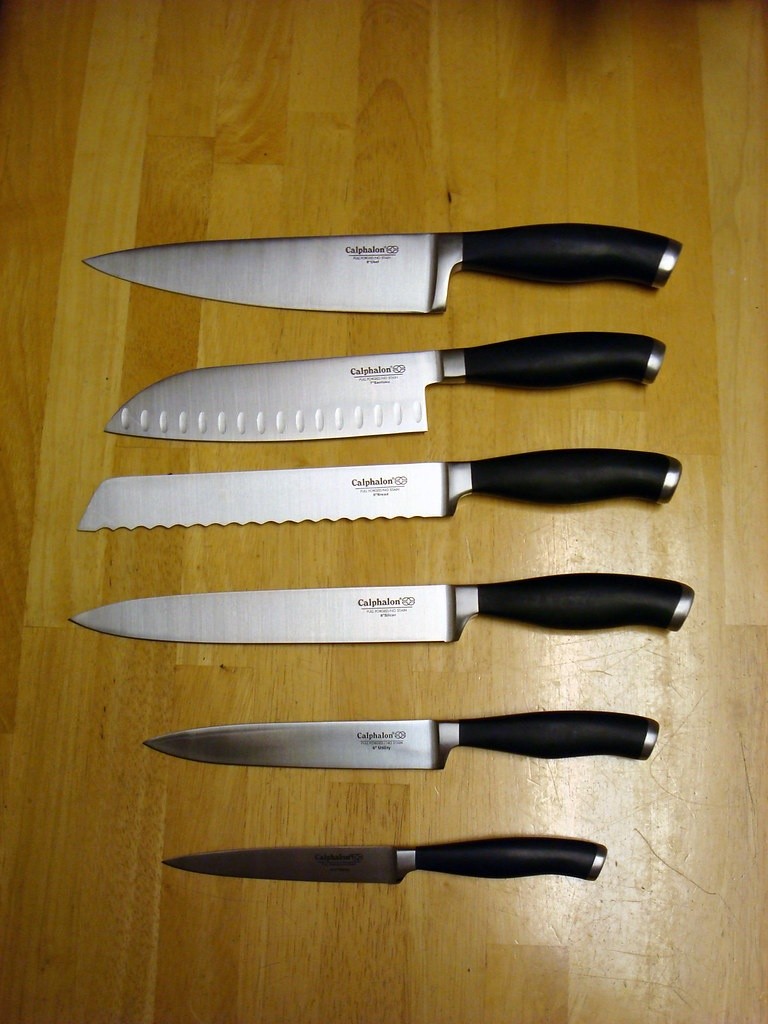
left=141, top=710, right=660, bottom=771
left=81, top=223, right=683, bottom=314
left=77, top=450, right=681, bottom=531
left=161, top=837, right=607, bottom=884
left=69, top=572, right=694, bottom=643
left=103, top=332, right=665, bottom=441
